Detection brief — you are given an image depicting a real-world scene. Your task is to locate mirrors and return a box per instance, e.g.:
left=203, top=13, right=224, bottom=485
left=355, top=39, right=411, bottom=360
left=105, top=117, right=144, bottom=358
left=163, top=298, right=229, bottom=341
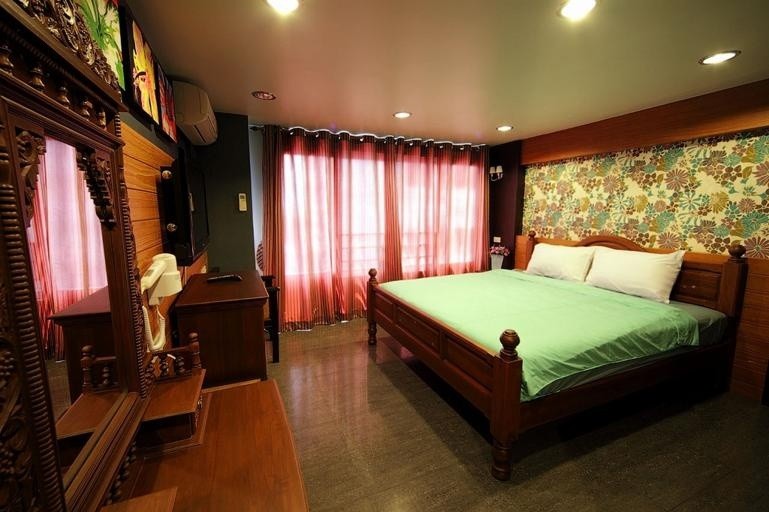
left=0, top=0, right=156, bottom=512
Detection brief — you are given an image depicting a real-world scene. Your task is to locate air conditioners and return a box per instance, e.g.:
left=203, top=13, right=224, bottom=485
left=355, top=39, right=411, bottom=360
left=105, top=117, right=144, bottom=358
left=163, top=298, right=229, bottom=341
left=172, top=80, right=219, bottom=146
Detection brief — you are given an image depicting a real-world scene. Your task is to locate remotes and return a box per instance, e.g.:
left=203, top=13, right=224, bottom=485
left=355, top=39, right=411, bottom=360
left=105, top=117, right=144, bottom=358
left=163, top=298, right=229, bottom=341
left=208, top=274, right=243, bottom=281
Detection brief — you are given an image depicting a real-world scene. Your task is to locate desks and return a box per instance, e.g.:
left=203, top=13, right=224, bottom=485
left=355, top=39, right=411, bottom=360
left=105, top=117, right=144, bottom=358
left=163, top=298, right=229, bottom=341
left=47, top=286, right=121, bottom=405
left=175, top=269, right=270, bottom=388
left=99, top=369, right=310, bottom=512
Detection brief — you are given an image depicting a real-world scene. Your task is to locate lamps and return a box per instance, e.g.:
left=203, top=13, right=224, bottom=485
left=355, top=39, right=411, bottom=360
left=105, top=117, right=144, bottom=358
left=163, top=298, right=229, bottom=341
left=490, top=165, right=503, bottom=181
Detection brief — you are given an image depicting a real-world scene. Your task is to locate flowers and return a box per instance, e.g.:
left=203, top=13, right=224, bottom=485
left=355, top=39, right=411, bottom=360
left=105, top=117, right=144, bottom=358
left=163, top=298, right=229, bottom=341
left=488, top=244, right=510, bottom=256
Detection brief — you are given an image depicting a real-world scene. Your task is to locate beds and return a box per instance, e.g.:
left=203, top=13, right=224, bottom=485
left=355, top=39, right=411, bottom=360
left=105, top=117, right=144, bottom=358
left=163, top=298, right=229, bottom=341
left=366, top=230, right=746, bottom=481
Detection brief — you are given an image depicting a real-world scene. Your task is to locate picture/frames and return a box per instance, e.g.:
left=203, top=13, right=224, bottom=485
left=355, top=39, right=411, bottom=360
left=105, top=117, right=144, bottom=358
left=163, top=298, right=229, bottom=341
left=72, top=0, right=128, bottom=93
left=155, top=55, right=179, bottom=144
left=124, top=3, right=159, bottom=126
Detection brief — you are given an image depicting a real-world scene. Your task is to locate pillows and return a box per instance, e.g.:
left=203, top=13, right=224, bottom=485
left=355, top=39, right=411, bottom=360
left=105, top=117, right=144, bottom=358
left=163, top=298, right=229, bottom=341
left=523, top=243, right=594, bottom=283
left=584, top=246, right=687, bottom=305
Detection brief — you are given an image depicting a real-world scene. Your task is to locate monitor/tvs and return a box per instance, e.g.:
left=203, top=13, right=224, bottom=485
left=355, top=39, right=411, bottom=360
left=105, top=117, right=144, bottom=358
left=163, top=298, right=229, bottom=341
left=187, top=166, right=211, bottom=262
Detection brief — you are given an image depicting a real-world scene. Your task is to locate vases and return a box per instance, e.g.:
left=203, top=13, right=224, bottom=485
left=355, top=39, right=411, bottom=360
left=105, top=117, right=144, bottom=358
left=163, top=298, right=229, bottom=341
left=491, top=255, right=504, bottom=270
